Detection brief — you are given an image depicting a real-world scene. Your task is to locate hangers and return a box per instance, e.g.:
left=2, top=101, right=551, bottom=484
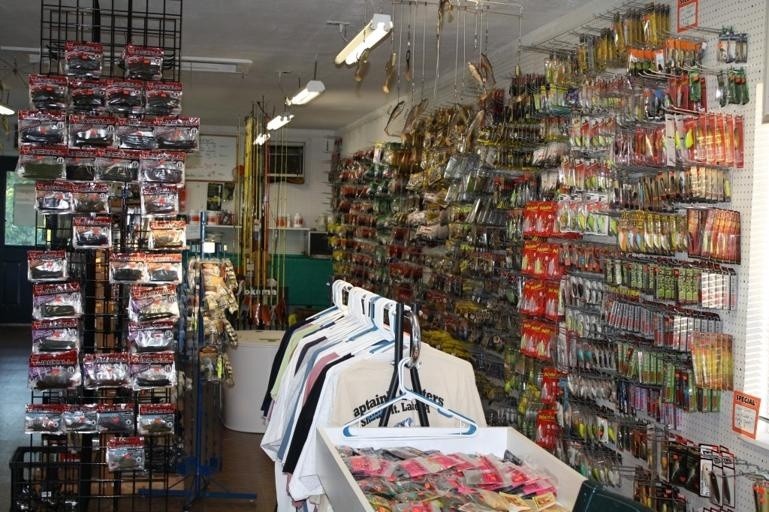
left=341, top=357, right=479, bottom=440
left=305, top=279, right=424, bottom=363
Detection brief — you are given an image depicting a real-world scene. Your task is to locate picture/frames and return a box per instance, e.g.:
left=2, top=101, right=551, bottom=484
left=182, top=133, right=240, bottom=183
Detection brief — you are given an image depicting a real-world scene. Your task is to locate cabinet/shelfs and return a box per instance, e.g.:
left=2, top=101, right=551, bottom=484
left=310, top=424, right=645, bottom=511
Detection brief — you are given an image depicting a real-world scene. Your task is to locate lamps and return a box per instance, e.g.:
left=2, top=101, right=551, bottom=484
left=285, top=61, right=325, bottom=106
left=265, top=97, right=295, bottom=131
left=326, top=14, right=393, bottom=65
left=0, top=83, right=15, bottom=115
left=173, top=56, right=254, bottom=79
left=253, top=132, right=270, bottom=146
